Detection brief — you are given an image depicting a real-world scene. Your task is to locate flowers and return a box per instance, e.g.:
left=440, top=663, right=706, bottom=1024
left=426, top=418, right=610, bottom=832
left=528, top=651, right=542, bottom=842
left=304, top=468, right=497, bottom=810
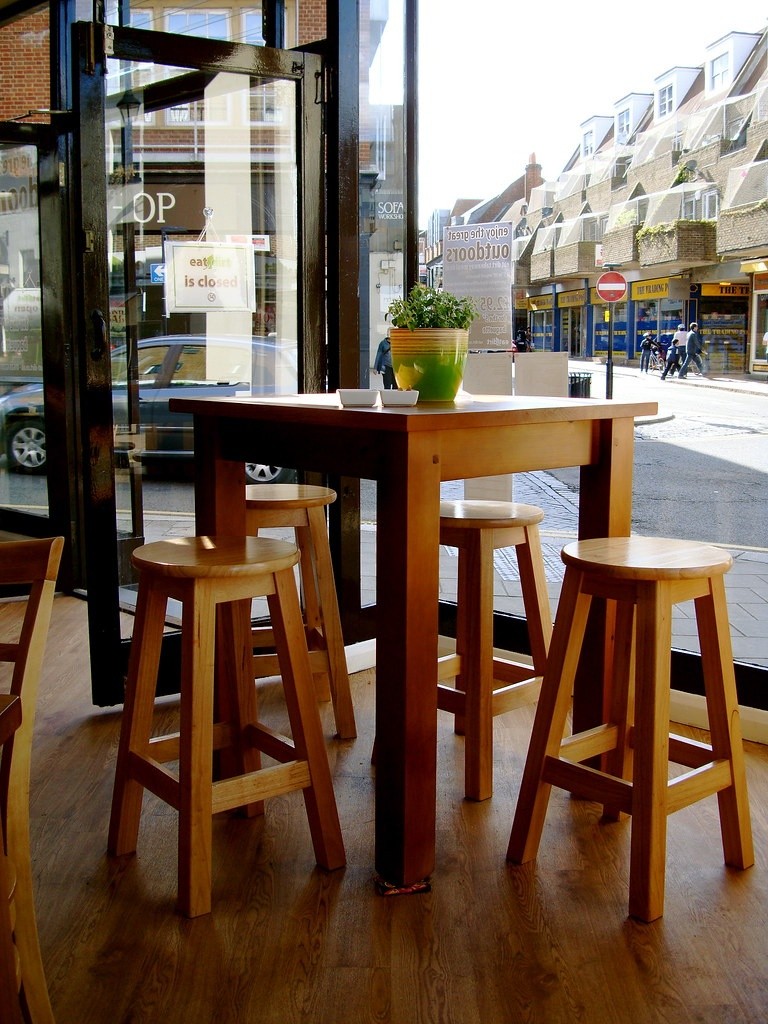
left=384, top=280, right=480, bottom=333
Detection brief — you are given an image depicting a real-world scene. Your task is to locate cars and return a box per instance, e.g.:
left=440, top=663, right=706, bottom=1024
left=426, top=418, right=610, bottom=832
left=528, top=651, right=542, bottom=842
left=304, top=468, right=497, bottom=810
left=0, top=333, right=323, bottom=486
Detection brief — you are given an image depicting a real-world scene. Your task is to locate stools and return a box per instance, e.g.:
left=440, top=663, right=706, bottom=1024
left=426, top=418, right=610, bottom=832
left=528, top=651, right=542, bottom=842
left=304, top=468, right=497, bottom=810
left=369, top=500, right=551, bottom=802
left=107, top=537, right=346, bottom=916
left=240, top=484, right=357, bottom=739
left=507, top=537, right=755, bottom=922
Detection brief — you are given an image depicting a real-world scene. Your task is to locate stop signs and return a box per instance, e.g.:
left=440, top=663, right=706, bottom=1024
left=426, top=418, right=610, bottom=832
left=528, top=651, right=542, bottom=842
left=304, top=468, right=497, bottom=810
left=595, top=272, right=628, bottom=303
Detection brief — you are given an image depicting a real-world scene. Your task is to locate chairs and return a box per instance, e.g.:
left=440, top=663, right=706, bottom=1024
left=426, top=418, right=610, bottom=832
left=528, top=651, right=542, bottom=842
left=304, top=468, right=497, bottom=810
left=0, top=537, right=66, bottom=1024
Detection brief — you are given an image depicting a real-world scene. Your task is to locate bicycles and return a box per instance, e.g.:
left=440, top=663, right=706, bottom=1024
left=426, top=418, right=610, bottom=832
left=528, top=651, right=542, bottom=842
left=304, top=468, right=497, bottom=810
left=689, top=354, right=703, bottom=375
left=648, top=347, right=665, bottom=374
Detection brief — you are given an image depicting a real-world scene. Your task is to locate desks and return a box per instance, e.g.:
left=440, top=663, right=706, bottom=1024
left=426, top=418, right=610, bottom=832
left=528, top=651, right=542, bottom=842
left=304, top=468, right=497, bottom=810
left=167, top=397, right=661, bottom=891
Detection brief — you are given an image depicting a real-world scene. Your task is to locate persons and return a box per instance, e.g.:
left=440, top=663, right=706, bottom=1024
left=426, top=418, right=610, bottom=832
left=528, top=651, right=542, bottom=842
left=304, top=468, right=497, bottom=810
left=373, top=327, right=399, bottom=390
left=640, top=332, right=660, bottom=372
left=651, top=342, right=666, bottom=362
left=525, top=327, right=531, bottom=352
left=668, top=324, right=689, bottom=378
left=660, top=339, right=681, bottom=380
left=516, top=326, right=525, bottom=352
left=678, top=323, right=708, bottom=379
left=762, top=331, right=768, bottom=384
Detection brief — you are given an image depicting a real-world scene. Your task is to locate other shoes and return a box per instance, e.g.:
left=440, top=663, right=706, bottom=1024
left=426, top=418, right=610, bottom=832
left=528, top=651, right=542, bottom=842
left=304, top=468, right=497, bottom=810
left=678, top=376, right=687, bottom=379
left=661, top=376, right=665, bottom=380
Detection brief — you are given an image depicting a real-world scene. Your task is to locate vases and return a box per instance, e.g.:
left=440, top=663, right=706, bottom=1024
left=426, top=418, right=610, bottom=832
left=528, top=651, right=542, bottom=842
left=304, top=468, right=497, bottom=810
left=391, top=327, right=469, bottom=402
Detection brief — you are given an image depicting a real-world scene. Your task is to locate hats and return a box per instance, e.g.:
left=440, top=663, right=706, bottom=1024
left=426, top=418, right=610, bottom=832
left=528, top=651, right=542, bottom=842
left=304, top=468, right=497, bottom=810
left=678, top=324, right=685, bottom=329
left=643, top=332, right=651, bottom=337
